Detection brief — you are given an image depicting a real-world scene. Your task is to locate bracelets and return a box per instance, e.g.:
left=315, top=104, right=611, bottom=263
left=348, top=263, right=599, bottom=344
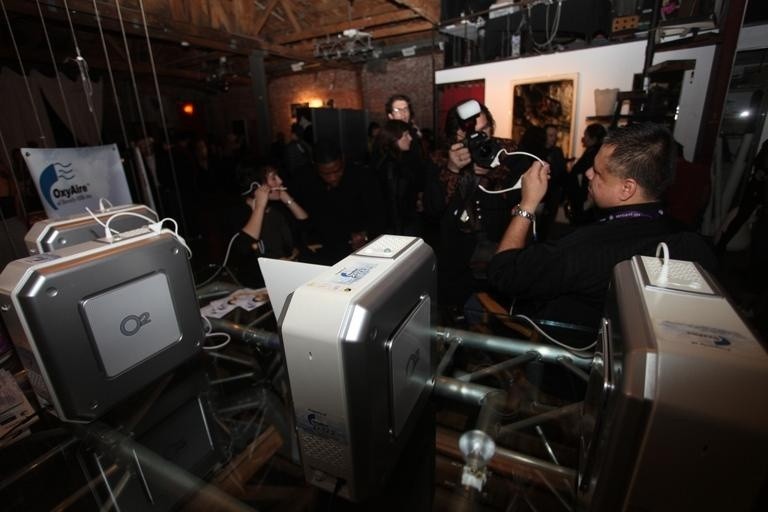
left=512, top=202, right=537, bottom=225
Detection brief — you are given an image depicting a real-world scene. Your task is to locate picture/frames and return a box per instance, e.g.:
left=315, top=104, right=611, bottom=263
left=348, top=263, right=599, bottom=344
left=508, top=76, right=576, bottom=172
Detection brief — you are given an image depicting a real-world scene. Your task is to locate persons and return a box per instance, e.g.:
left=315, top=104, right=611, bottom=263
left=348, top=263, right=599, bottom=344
left=663, top=138, right=711, bottom=224
left=354, top=93, right=609, bottom=294
left=717, top=137, right=766, bottom=260
left=486, top=121, right=713, bottom=413
left=129, top=129, right=378, bottom=291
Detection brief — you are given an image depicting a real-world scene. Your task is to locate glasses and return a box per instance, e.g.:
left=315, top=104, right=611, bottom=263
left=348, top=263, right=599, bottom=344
left=392, top=107, right=410, bottom=113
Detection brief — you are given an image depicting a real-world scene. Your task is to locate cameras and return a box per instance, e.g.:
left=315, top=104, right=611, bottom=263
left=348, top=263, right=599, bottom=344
left=456, top=98, right=502, bottom=169
